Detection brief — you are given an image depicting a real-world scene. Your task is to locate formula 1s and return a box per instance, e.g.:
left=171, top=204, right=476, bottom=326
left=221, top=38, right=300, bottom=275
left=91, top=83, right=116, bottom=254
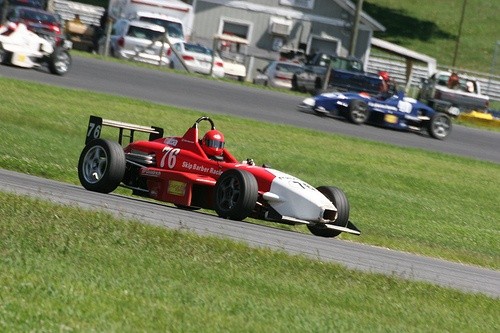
left=77, top=115, right=362, bottom=237
left=296, top=75, right=460, bottom=140
left=0, top=20, right=73, bottom=75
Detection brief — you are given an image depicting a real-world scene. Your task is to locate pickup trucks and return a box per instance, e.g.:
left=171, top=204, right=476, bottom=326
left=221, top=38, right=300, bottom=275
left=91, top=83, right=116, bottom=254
left=417, top=70, right=491, bottom=115
left=291, top=53, right=388, bottom=99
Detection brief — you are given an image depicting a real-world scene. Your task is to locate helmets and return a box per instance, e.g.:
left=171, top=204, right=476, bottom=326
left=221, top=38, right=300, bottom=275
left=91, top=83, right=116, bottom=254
left=201, top=130, right=224, bottom=156
left=377, top=71, right=390, bottom=82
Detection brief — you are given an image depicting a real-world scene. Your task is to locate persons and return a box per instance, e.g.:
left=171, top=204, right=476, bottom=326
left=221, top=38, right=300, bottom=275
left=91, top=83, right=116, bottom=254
left=1, top=21, right=17, bottom=36
left=373, top=80, right=393, bottom=101
left=200, top=129, right=226, bottom=163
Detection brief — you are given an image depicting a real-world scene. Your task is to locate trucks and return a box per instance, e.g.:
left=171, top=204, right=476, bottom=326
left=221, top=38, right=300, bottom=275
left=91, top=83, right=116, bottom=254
left=108, top=0, right=195, bottom=48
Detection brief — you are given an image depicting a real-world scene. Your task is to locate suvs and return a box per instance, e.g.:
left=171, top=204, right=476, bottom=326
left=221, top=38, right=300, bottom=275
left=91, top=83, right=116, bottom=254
left=97, top=18, right=173, bottom=66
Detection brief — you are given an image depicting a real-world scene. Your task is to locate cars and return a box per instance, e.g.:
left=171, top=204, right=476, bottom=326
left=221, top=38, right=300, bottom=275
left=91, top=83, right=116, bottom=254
left=7, top=6, right=63, bottom=47
left=254, top=61, right=304, bottom=89
left=167, top=41, right=225, bottom=80
